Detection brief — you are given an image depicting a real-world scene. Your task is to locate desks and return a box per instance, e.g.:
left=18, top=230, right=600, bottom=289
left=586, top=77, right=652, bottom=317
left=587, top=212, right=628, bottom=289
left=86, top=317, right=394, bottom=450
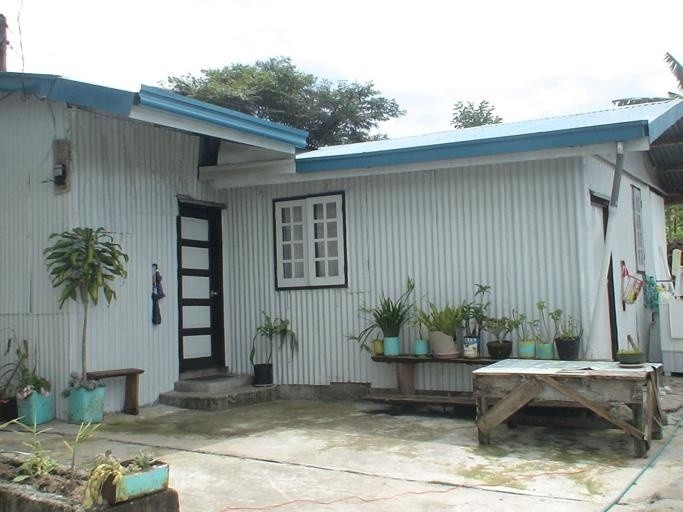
left=472, top=356, right=669, bottom=459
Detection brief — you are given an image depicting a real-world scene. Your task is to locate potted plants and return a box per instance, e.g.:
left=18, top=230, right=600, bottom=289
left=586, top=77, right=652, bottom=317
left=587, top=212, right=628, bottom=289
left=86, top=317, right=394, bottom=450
left=81, top=452, right=170, bottom=506
left=342, top=274, right=584, bottom=361
left=0, top=414, right=180, bottom=512
left=249, top=310, right=300, bottom=386
left=0, top=326, right=56, bottom=426
left=616, top=348, right=644, bottom=364
left=42, top=225, right=129, bottom=426
